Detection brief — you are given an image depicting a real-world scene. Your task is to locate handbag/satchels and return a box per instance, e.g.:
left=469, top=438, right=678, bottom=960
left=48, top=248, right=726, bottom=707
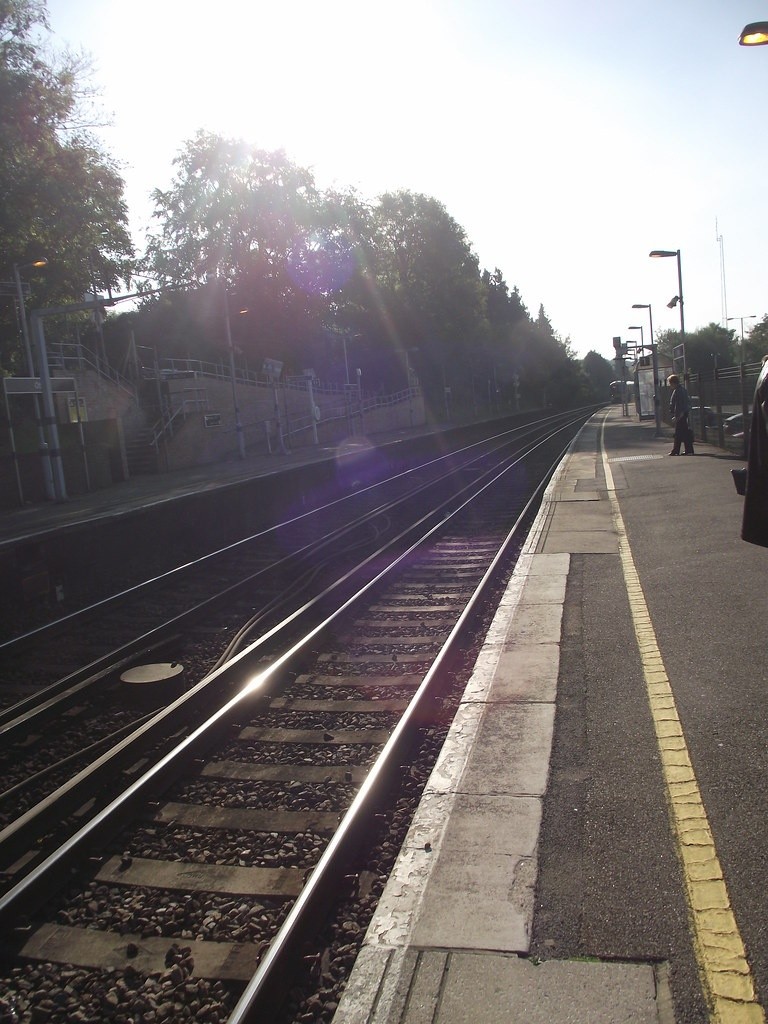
left=684, top=416, right=694, bottom=447
left=730, top=467, right=747, bottom=495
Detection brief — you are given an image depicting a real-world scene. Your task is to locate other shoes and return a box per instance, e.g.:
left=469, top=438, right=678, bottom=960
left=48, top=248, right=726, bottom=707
left=681, top=448, right=694, bottom=456
left=667, top=450, right=680, bottom=456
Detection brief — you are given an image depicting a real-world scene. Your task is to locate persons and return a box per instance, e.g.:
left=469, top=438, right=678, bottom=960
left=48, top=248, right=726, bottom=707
left=667, top=375, right=694, bottom=456
left=738, top=355, right=768, bottom=549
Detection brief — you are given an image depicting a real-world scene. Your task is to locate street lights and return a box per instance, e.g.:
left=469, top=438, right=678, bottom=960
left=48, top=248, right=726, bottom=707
left=727, top=316, right=757, bottom=461
left=224, top=305, right=250, bottom=459
left=11, top=255, right=56, bottom=503
left=649, top=249, right=688, bottom=392
left=626, top=341, right=637, bottom=355
left=632, top=303, right=661, bottom=432
left=628, top=326, right=645, bottom=357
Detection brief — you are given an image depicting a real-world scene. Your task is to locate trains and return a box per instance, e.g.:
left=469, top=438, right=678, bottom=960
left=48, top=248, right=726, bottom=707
left=610, top=380, right=642, bottom=404
